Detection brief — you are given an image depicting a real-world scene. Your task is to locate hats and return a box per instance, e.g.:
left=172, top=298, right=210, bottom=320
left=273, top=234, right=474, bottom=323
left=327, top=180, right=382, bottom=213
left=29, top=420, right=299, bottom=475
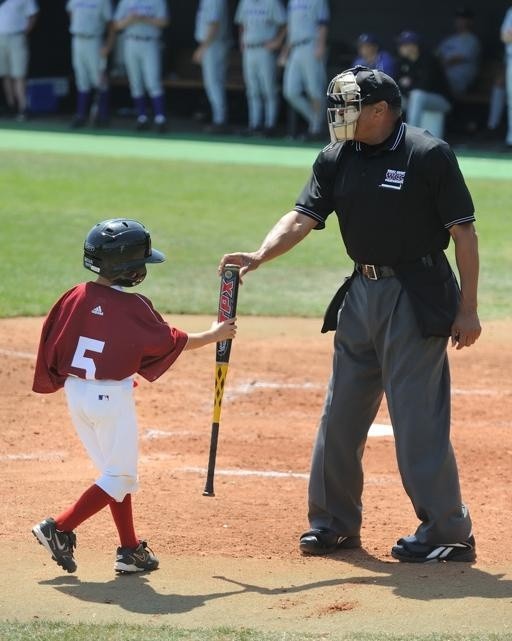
left=392, top=29, right=420, bottom=45
left=327, top=69, right=403, bottom=108
left=355, top=32, right=376, bottom=45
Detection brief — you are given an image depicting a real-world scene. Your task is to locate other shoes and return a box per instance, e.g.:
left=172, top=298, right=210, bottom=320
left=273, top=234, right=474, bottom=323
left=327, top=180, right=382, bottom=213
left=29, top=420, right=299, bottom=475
left=16, top=112, right=27, bottom=123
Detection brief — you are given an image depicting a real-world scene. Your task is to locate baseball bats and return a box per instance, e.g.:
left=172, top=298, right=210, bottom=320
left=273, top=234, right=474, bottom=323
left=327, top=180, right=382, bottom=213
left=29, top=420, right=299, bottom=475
left=204, top=264, right=239, bottom=497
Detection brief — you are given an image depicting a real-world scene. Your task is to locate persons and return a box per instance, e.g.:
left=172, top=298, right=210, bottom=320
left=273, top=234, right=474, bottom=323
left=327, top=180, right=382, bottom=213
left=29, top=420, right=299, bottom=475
left=213, top=63, right=482, bottom=564
left=1, top=1, right=511, bottom=148
left=30, top=219, right=237, bottom=574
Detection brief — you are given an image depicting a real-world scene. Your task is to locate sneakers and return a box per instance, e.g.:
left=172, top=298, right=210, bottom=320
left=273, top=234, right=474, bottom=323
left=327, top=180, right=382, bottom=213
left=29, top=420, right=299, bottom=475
left=70, top=113, right=89, bottom=128
left=389, top=532, right=478, bottom=567
left=133, top=114, right=150, bottom=129
left=154, top=114, right=168, bottom=133
left=112, top=540, right=161, bottom=575
left=298, top=531, right=362, bottom=555
left=31, top=517, right=79, bottom=577
left=206, top=115, right=326, bottom=138
left=93, top=113, right=109, bottom=130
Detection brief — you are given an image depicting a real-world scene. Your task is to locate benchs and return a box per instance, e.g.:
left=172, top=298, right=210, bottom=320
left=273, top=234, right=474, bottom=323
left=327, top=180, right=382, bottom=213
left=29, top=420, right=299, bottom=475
left=115, top=73, right=511, bottom=140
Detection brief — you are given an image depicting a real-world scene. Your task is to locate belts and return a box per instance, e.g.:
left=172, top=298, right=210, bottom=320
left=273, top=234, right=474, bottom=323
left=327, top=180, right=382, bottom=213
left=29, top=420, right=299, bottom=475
left=350, top=251, right=445, bottom=283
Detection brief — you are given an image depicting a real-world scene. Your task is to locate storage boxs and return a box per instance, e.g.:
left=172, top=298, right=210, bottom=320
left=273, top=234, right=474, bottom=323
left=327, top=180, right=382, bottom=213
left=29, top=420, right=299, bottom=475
left=26, top=77, right=67, bottom=113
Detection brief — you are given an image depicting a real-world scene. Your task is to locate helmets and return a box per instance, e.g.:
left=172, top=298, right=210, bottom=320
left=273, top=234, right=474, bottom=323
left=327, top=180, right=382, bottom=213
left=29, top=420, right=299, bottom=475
left=82, top=217, right=168, bottom=280
